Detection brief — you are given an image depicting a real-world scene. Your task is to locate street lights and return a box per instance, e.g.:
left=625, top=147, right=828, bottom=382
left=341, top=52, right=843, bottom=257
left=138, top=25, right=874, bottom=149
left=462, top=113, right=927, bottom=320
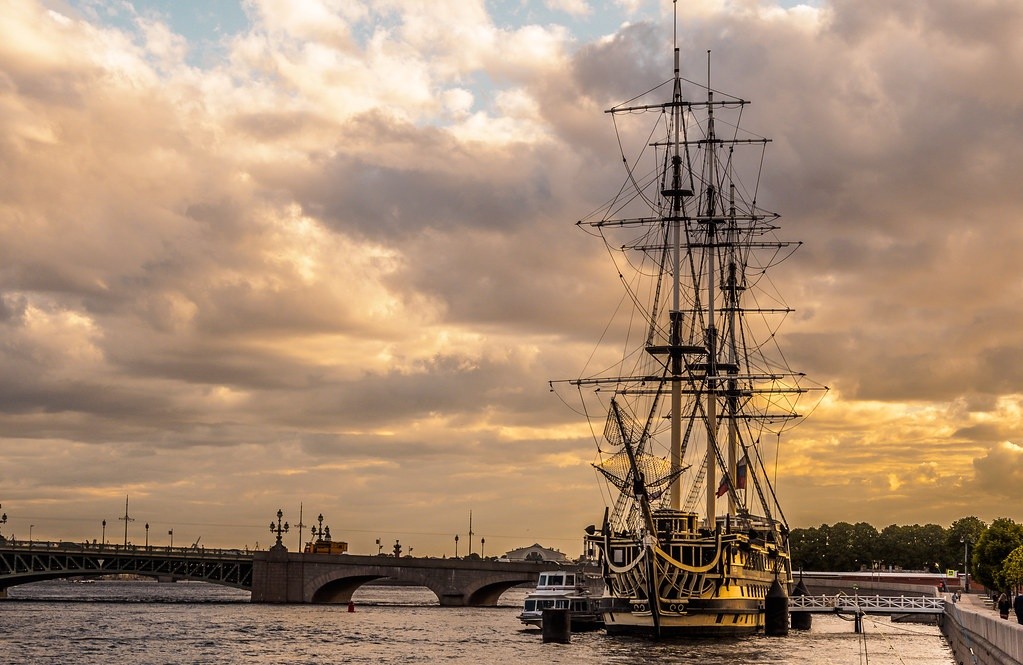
left=102, top=520, right=106, bottom=543
left=145, top=523, right=149, bottom=546
left=481, top=537, right=485, bottom=561
left=311, top=514, right=329, bottom=541
left=455, top=535, right=459, bottom=558
left=29, top=524, right=35, bottom=542
left=270, top=510, right=289, bottom=552
left=960, top=534, right=968, bottom=594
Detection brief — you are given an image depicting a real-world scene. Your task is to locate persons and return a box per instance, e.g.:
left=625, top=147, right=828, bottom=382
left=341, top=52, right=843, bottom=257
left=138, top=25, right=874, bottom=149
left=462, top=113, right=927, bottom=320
left=957, top=588, right=962, bottom=601
left=1013, top=596, right=1023, bottom=625
left=127, top=542, right=132, bottom=550
left=202, top=545, right=204, bottom=553
left=992, top=592, right=998, bottom=611
left=939, top=581, right=943, bottom=591
left=93, top=540, right=96, bottom=548
left=997, top=593, right=1010, bottom=620
left=86, top=540, right=89, bottom=548
left=952, top=593, right=958, bottom=603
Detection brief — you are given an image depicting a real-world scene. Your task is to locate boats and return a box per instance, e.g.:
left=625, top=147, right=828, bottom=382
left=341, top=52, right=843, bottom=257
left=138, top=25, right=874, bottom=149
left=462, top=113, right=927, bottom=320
left=516, top=570, right=604, bottom=630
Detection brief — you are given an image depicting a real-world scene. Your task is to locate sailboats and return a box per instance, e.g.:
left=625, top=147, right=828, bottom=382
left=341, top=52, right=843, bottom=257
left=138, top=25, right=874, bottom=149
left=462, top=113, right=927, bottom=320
left=543, top=2, right=835, bottom=645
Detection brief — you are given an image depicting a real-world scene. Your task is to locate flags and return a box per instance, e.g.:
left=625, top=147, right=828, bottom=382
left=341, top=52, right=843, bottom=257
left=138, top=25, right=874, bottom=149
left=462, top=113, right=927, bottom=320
left=717, top=456, right=746, bottom=499
